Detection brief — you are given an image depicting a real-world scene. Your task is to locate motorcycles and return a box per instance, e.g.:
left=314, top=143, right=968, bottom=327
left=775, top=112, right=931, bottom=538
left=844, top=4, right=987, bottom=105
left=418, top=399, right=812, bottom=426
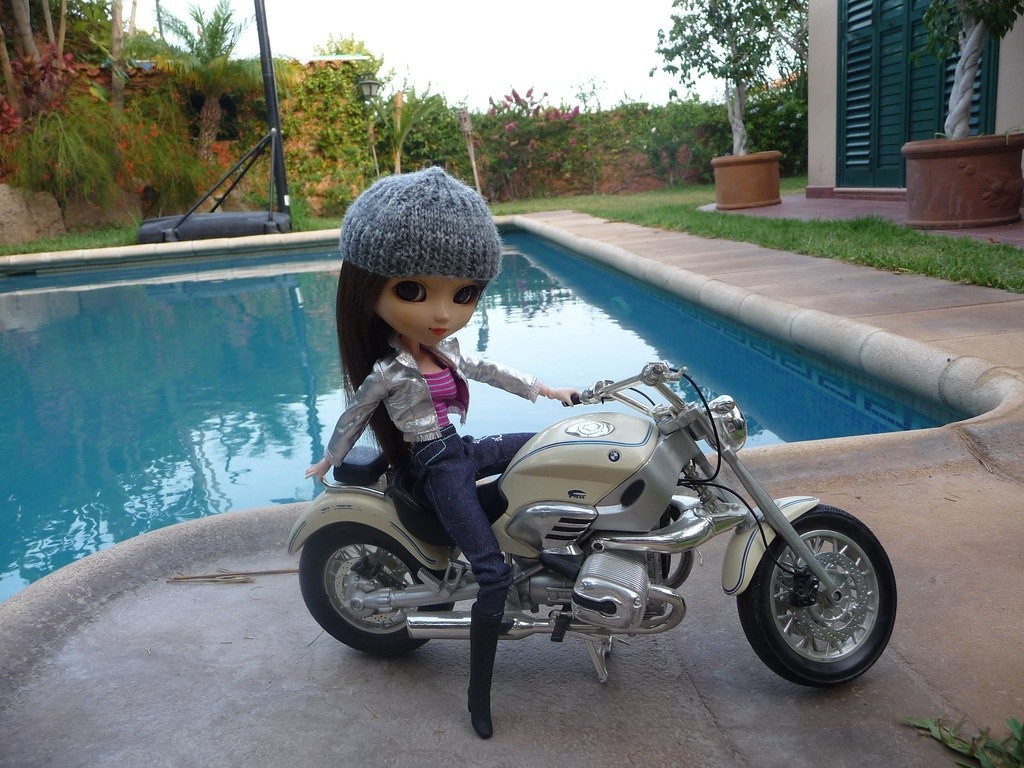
left=289, top=362, right=898, bottom=688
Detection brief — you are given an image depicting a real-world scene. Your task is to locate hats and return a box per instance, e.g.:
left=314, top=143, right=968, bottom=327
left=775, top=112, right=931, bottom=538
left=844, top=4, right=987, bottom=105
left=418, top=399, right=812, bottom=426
left=339, top=166, right=502, bottom=282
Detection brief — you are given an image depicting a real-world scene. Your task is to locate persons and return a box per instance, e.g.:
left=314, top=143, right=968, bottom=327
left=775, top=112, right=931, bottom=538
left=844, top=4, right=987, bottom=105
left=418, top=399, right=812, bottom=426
left=305, top=166, right=579, bottom=739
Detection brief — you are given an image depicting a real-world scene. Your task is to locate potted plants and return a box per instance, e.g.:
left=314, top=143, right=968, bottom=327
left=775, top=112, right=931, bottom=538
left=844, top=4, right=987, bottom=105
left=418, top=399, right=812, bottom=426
left=898, top=0, right=1024, bottom=229
left=648, top=0, right=809, bottom=211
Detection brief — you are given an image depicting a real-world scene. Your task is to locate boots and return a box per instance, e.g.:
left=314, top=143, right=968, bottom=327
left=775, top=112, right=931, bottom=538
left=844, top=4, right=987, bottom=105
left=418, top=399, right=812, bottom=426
left=467, top=603, right=504, bottom=738
